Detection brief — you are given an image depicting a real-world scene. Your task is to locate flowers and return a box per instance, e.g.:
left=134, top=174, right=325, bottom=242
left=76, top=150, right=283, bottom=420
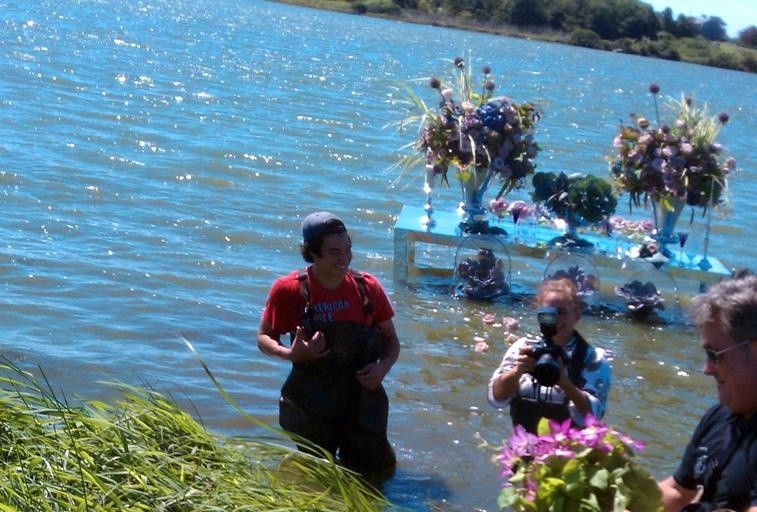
left=608, top=215, right=653, bottom=244
left=525, top=201, right=552, bottom=225
left=378, top=48, right=547, bottom=201
left=507, top=201, right=530, bottom=222
left=489, top=197, right=508, bottom=217
left=604, top=78, right=740, bottom=245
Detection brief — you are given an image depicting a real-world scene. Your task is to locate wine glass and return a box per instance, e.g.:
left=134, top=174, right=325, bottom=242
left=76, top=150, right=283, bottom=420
left=677, top=233, right=690, bottom=261
left=512, top=207, right=522, bottom=237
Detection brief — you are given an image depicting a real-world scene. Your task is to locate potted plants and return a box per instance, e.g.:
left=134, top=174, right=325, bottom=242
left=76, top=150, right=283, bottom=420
left=526, top=172, right=617, bottom=235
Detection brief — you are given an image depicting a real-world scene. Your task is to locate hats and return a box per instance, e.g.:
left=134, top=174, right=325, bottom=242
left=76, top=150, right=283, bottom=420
left=303, top=219, right=346, bottom=240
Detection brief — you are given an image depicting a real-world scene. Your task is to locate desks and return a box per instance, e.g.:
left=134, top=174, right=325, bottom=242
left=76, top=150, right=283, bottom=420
left=393, top=203, right=732, bottom=289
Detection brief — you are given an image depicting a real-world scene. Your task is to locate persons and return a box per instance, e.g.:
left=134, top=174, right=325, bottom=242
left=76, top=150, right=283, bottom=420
left=257, top=211, right=400, bottom=484
left=486, top=278, right=611, bottom=479
left=656, top=275, right=756, bottom=511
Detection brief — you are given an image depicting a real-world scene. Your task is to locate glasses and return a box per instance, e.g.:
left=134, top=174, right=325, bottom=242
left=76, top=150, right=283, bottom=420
left=705, top=340, right=752, bottom=365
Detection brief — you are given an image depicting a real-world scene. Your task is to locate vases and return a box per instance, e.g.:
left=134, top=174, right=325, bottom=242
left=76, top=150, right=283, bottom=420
left=527, top=221, right=537, bottom=247
left=495, top=216, right=502, bottom=228
left=653, top=198, right=683, bottom=258
left=456, top=164, right=493, bottom=223
left=512, top=221, right=519, bottom=240
left=614, top=235, right=632, bottom=259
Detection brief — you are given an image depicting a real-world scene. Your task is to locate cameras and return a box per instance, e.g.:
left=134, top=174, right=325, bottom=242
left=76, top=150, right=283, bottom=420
left=524, top=307, right=569, bottom=387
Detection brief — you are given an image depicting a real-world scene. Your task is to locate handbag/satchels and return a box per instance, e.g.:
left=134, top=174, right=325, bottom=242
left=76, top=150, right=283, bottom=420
left=680, top=501, right=716, bottom=512
left=299, top=274, right=383, bottom=376
left=278, top=383, right=352, bottom=447
left=510, top=385, right=569, bottom=435
